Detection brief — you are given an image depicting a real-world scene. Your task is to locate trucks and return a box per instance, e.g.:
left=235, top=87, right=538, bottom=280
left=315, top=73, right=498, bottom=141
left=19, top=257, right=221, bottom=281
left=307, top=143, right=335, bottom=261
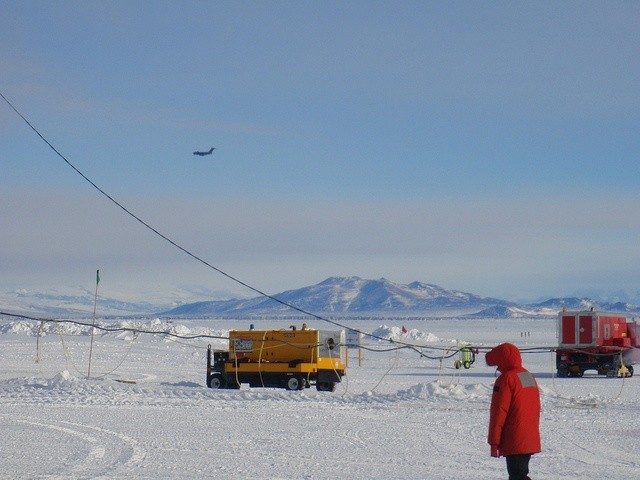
left=206, top=324, right=347, bottom=392
left=550, top=305, right=639, bottom=378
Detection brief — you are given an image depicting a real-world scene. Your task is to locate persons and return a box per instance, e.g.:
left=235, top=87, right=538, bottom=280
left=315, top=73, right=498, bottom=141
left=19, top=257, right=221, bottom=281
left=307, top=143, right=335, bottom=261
left=485, top=343, right=542, bottom=480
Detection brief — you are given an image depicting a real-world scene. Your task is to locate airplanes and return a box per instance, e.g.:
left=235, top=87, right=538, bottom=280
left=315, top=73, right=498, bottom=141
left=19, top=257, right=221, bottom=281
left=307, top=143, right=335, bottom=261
left=192, top=146, right=214, bottom=155
